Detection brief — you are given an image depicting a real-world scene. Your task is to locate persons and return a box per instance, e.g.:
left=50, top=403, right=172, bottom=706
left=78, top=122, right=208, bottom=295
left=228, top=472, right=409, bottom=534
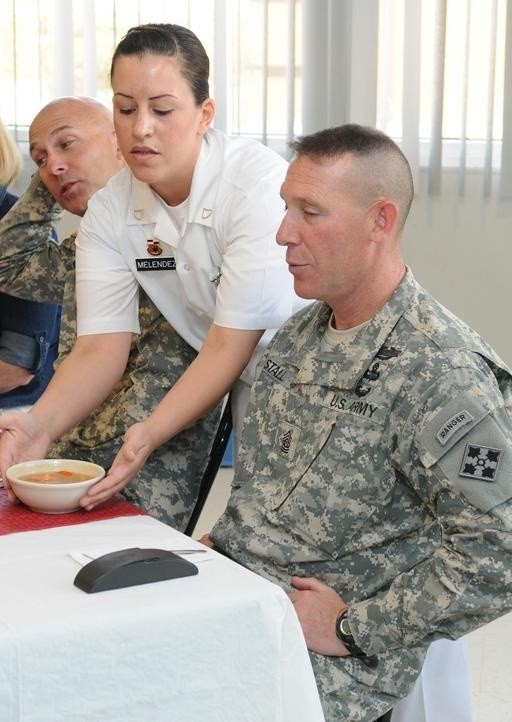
left=0, top=115, right=60, bottom=416
left=0, top=23, right=316, bottom=512
left=197, top=124, right=512, bottom=722
left=0, top=96, right=223, bottom=534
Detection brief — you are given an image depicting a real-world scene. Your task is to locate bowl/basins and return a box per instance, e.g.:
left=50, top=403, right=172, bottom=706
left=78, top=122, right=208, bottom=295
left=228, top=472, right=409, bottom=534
left=4, top=457, right=106, bottom=516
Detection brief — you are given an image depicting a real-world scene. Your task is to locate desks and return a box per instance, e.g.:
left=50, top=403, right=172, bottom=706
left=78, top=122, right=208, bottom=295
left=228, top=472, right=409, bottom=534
left=0, top=465, right=333, bottom=722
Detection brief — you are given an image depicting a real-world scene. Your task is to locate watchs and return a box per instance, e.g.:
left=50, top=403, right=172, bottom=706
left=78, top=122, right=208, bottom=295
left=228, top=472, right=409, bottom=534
left=334, top=608, right=366, bottom=660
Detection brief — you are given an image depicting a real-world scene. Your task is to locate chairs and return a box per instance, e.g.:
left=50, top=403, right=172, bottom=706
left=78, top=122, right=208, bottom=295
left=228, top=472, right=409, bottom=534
left=388, top=683, right=426, bottom=721
left=0, top=288, right=61, bottom=412
left=181, top=387, right=235, bottom=537
left=421, top=611, right=511, bottom=721
